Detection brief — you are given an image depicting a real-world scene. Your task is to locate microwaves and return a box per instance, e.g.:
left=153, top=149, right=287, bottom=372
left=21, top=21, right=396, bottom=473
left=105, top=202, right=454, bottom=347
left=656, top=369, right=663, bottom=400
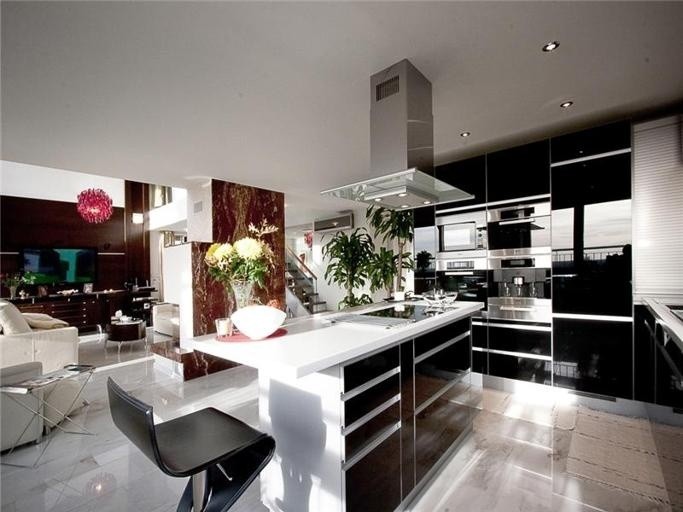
left=441, top=223, right=476, bottom=252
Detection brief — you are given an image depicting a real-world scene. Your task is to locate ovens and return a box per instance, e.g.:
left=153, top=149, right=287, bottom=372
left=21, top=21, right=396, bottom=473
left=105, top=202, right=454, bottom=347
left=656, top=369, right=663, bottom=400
left=486, top=201, right=552, bottom=268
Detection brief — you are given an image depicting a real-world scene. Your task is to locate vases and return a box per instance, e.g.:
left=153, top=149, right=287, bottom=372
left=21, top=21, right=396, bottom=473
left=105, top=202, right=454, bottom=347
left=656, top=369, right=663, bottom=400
left=229, top=280, right=254, bottom=309
left=9, top=286, right=16, bottom=300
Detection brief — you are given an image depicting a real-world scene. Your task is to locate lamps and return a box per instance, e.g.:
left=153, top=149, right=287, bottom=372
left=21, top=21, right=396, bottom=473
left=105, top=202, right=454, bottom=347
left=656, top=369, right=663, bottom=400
left=76, top=186, right=113, bottom=225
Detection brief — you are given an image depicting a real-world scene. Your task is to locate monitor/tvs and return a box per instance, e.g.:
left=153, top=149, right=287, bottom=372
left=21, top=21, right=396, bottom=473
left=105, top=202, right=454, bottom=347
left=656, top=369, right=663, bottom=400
left=19, top=247, right=99, bottom=287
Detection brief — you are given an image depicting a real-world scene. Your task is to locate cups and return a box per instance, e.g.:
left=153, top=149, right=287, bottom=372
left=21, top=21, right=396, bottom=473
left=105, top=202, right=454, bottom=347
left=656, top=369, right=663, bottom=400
left=215, top=318, right=233, bottom=337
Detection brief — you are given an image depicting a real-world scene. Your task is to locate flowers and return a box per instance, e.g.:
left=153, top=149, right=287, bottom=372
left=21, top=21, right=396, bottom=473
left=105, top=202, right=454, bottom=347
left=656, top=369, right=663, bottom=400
left=204, top=237, right=275, bottom=289
left=0, top=272, right=26, bottom=285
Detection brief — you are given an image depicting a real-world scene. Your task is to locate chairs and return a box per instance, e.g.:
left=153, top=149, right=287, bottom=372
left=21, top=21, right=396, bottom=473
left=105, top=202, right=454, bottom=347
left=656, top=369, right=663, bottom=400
left=107, top=377, right=276, bottom=512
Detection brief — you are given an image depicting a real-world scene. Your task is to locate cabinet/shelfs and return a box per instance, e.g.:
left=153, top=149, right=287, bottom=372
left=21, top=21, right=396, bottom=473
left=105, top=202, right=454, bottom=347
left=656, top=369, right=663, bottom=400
left=7, top=292, right=136, bottom=333
left=179, top=303, right=486, bottom=512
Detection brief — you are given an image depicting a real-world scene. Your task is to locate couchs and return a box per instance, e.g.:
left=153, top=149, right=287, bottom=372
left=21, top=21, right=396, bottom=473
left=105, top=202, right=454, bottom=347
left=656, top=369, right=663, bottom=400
left=0, top=302, right=78, bottom=374
left=152, top=303, right=179, bottom=337
